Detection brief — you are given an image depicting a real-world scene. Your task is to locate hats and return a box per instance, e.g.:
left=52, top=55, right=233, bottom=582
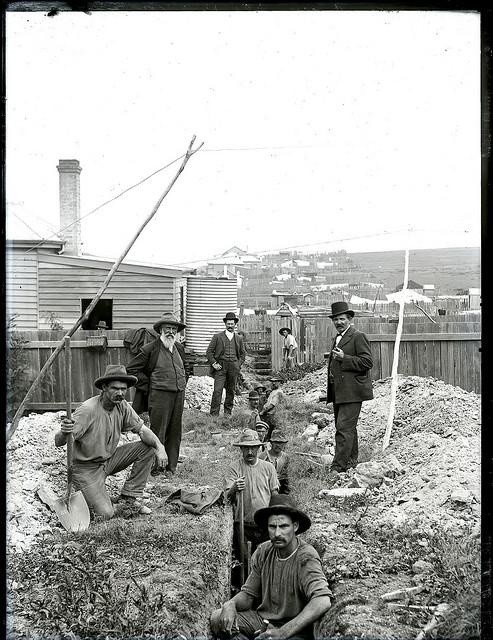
left=246, top=376, right=283, bottom=400
left=232, top=427, right=266, bottom=447
left=279, top=327, right=292, bottom=336
left=222, top=312, right=239, bottom=324
left=94, top=364, right=139, bottom=390
left=153, top=312, right=186, bottom=334
left=327, top=301, right=355, bottom=320
left=269, top=429, right=289, bottom=444
left=255, top=420, right=270, bottom=430
left=253, top=494, right=312, bottom=536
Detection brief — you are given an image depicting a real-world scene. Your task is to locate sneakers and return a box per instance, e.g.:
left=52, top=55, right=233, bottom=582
left=117, top=494, right=152, bottom=516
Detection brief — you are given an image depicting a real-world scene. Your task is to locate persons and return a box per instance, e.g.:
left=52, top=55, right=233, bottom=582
left=222, top=382, right=293, bottom=591
left=278, top=327, right=298, bottom=369
left=125, top=311, right=191, bottom=477
left=53, top=364, right=168, bottom=520
left=207, top=493, right=335, bottom=639
left=324, top=301, right=375, bottom=476
left=205, top=313, right=246, bottom=417
left=94, top=321, right=108, bottom=331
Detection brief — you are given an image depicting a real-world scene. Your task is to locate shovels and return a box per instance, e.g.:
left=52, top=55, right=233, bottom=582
left=54, top=337, right=91, bottom=534
